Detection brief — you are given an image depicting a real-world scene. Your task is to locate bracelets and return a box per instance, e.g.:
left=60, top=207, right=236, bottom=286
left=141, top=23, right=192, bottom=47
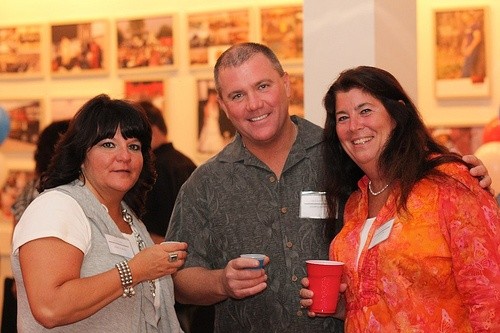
left=115, top=260, right=135, bottom=299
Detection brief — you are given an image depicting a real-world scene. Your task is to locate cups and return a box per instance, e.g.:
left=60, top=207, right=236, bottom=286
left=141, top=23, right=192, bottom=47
left=160, top=241, right=178, bottom=246
left=306, top=260, right=346, bottom=316
left=240, top=254, right=265, bottom=270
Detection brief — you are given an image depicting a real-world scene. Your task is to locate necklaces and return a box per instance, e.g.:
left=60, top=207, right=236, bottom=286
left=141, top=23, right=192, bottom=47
left=369, top=181, right=388, bottom=195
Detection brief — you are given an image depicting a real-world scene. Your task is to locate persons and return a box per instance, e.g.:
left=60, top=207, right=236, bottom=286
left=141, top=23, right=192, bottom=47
left=300, top=65, right=500, bottom=333
left=11, top=120, right=70, bottom=223
left=199, top=90, right=223, bottom=154
left=11, top=94, right=188, bottom=333
left=164, top=42, right=492, bottom=333
left=461, top=12, right=482, bottom=78
left=139, top=101, right=197, bottom=244
left=55, top=37, right=101, bottom=71
left=124, top=31, right=160, bottom=69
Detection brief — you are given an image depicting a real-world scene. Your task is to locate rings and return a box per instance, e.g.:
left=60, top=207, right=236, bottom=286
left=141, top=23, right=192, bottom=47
left=170, top=252, right=178, bottom=262
